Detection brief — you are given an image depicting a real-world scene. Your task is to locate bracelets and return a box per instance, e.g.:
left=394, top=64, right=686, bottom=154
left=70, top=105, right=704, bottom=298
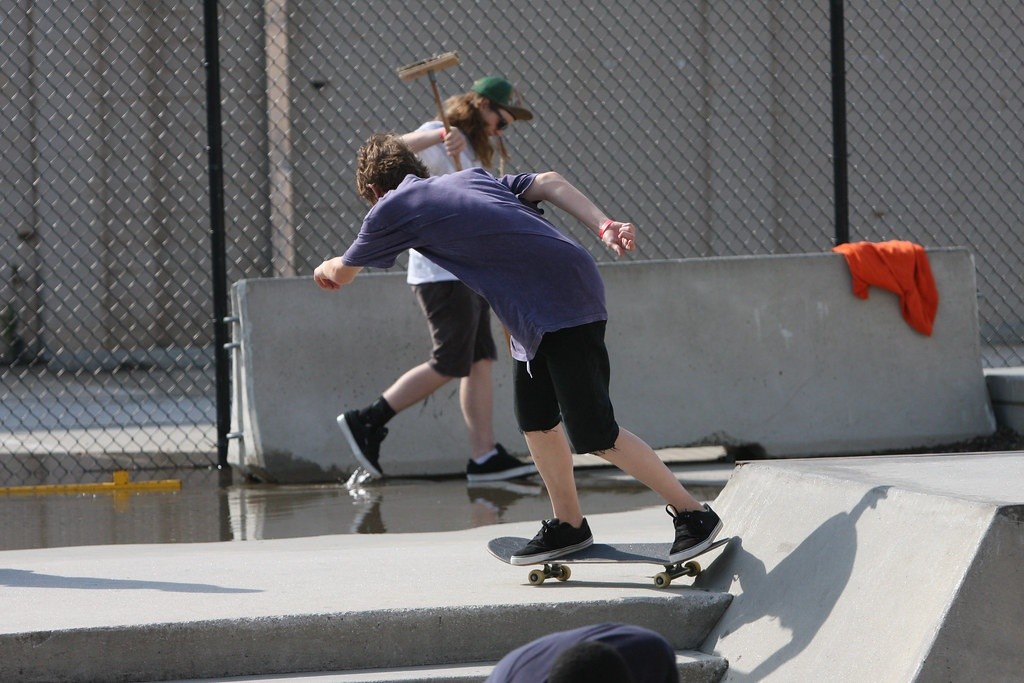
left=598, top=219, right=614, bottom=239
left=440, top=128, right=446, bottom=139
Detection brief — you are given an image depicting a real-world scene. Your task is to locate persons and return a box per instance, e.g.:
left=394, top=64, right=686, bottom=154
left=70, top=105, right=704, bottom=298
left=337, top=75, right=538, bottom=482
left=313, top=132, right=723, bottom=566
left=484, top=622, right=679, bottom=683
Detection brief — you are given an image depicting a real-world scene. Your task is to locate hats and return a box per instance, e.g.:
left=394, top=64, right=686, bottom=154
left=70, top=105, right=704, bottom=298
left=471, top=75, right=533, bottom=120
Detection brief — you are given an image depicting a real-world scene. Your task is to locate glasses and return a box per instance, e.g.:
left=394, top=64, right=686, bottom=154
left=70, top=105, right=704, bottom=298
left=490, top=102, right=509, bottom=131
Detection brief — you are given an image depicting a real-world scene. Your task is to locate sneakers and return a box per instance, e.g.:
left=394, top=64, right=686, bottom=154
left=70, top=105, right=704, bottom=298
left=466, top=442, right=539, bottom=482
left=336, top=409, right=388, bottom=478
left=510, top=516, right=594, bottom=565
left=666, top=503, right=724, bottom=563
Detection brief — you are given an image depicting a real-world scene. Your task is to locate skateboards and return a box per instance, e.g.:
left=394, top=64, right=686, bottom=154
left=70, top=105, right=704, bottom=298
left=485, top=535, right=731, bottom=590
